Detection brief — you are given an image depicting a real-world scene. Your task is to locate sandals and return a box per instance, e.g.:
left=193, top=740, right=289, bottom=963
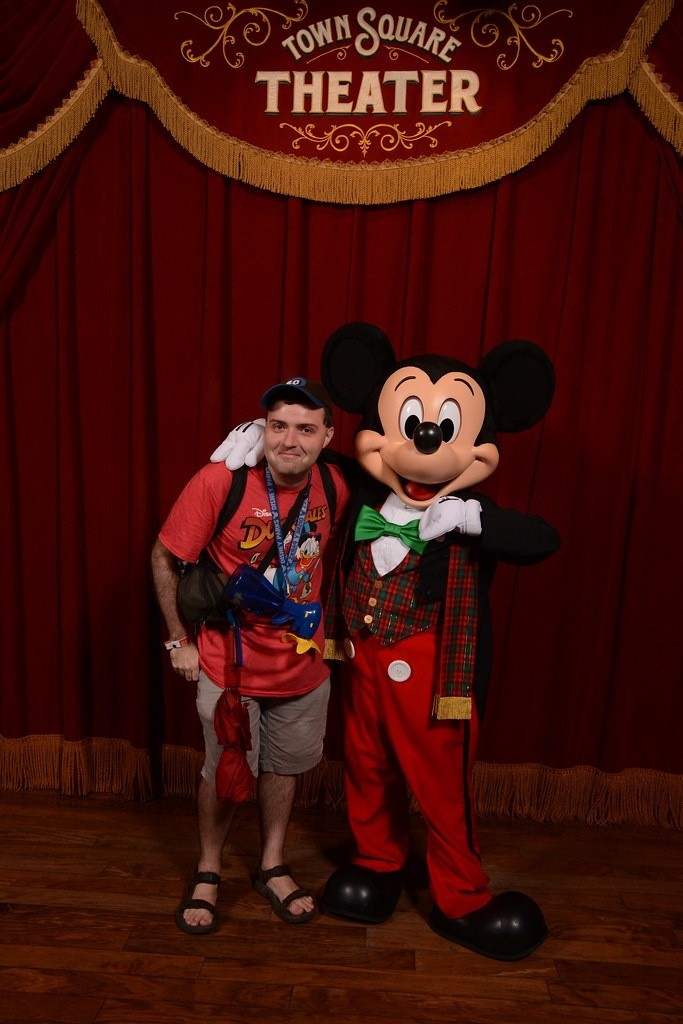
left=177, top=866, right=220, bottom=933
left=254, top=859, right=316, bottom=922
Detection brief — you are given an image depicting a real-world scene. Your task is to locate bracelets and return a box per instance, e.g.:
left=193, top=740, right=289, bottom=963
left=163, top=636, right=194, bottom=652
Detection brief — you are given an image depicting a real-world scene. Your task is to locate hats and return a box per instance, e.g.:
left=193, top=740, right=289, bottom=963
left=261, top=377, right=333, bottom=416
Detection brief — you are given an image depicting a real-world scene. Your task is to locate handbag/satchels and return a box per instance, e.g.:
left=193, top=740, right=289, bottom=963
left=178, top=549, right=229, bottom=628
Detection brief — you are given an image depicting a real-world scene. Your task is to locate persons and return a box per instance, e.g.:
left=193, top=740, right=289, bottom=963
left=146, top=377, right=349, bottom=936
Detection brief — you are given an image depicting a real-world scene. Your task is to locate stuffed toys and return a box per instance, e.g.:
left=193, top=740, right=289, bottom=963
left=207, top=319, right=560, bottom=960
